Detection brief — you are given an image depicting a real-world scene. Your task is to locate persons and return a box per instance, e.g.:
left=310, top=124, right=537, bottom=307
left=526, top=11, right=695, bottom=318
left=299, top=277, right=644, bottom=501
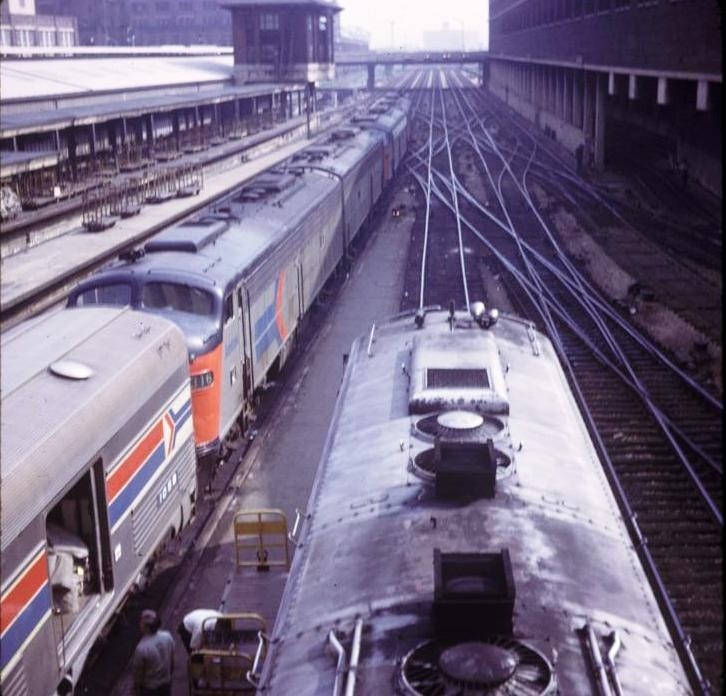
left=133, top=609, right=175, bottom=696
left=180, top=608, right=230, bottom=691
left=576, top=144, right=584, bottom=174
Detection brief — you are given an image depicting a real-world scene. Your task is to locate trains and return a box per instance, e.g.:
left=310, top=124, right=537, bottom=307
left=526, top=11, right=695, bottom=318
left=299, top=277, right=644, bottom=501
left=246, top=298, right=696, bottom=696
left=0, top=91, right=414, bottom=696
